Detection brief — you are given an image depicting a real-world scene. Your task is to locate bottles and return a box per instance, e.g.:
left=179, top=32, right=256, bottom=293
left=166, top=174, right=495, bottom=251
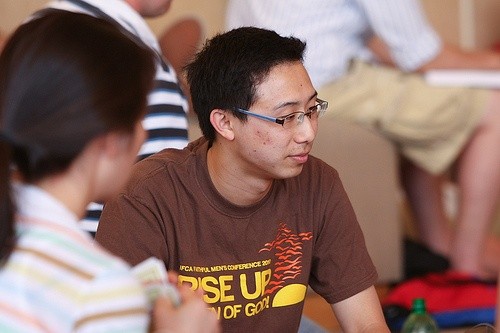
left=401, top=298, right=439, bottom=333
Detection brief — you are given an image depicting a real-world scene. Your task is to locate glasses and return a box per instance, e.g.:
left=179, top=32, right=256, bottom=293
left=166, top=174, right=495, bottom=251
left=228, top=97, right=329, bottom=129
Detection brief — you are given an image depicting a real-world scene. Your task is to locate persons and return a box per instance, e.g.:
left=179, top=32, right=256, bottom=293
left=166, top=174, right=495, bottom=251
left=0, top=8, right=216, bottom=333
left=23, top=0, right=201, bottom=237
left=223, top=0, right=500, bottom=282
left=95, top=27, right=390, bottom=333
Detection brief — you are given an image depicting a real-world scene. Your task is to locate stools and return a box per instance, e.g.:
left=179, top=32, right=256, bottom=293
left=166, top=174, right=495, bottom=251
left=310, top=119, right=405, bottom=281
